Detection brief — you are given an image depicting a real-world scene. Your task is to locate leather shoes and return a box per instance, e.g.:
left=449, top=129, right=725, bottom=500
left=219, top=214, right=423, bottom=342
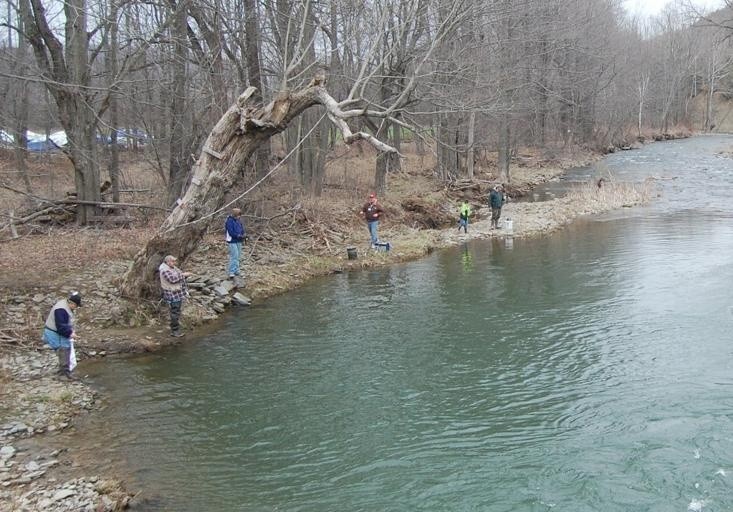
left=58, top=375, right=80, bottom=382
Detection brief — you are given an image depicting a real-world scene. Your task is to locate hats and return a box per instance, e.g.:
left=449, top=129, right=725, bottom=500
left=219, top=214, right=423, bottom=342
left=163, top=254, right=177, bottom=262
left=369, top=190, right=376, bottom=199
left=69, top=293, right=82, bottom=308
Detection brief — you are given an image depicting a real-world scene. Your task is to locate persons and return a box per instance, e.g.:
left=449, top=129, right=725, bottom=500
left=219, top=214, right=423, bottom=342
left=223, top=207, right=248, bottom=278
left=486, top=184, right=505, bottom=230
left=456, top=199, right=470, bottom=233
left=157, top=253, right=190, bottom=338
left=359, top=192, right=385, bottom=247
left=40, top=291, right=82, bottom=382
left=460, top=243, right=474, bottom=275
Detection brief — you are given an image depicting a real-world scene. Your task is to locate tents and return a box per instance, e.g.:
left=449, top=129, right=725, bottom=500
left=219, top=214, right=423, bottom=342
left=0, top=125, right=151, bottom=153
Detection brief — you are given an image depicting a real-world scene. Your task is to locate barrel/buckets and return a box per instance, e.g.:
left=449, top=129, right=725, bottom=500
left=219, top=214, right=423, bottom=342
left=347, top=248, right=359, bottom=260
left=504, top=220, right=514, bottom=233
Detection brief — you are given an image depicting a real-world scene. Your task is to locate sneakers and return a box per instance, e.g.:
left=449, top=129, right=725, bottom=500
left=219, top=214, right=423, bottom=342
left=169, top=331, right=185, bottom=337
